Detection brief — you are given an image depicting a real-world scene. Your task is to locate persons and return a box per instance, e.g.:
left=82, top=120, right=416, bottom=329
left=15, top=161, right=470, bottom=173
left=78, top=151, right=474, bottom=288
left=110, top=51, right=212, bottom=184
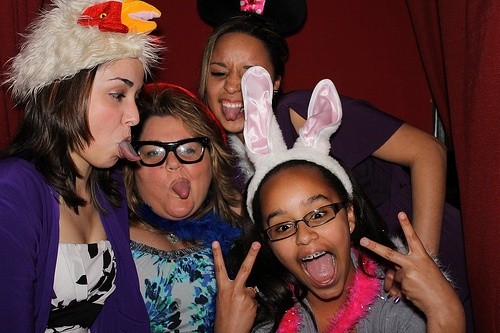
left=198, top=16, right=471, bottom=333
left=209, top=145, right=468, bottom=333
left=0, top=0, right=162, bottom=333
left=116, top=81, right=286, bottom=333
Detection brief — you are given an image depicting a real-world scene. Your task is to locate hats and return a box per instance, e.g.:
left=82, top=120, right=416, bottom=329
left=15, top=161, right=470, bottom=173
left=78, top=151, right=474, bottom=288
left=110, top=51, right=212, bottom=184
left=2, top=0, right=166, bottom=103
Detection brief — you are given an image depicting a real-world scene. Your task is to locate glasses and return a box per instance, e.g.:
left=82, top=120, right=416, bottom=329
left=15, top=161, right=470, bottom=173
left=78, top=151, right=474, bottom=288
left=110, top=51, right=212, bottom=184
left=260, top=200, right=350, bottom=242
left=130, top=137, right=210, bottom=167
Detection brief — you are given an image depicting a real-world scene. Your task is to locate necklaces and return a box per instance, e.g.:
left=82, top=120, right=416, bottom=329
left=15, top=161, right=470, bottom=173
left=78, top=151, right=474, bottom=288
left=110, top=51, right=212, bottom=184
left=137, top=218, right=181, bottom=246
